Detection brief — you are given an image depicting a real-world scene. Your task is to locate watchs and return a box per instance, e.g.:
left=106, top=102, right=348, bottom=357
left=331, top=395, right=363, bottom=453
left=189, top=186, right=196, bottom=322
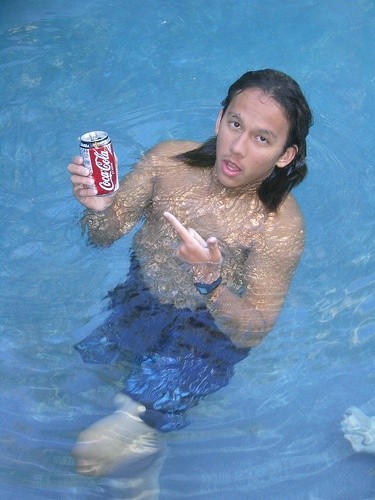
left=194, top=276, right=222, bottom=295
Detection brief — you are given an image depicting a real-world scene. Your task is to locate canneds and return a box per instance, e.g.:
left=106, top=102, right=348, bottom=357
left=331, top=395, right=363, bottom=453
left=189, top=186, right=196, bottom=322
left=80, top=130, right=120, bottom=196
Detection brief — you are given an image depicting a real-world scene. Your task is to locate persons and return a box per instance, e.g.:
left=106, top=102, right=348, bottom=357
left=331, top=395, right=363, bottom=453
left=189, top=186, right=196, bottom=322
left=68, top=68, right=313, bottom=499
